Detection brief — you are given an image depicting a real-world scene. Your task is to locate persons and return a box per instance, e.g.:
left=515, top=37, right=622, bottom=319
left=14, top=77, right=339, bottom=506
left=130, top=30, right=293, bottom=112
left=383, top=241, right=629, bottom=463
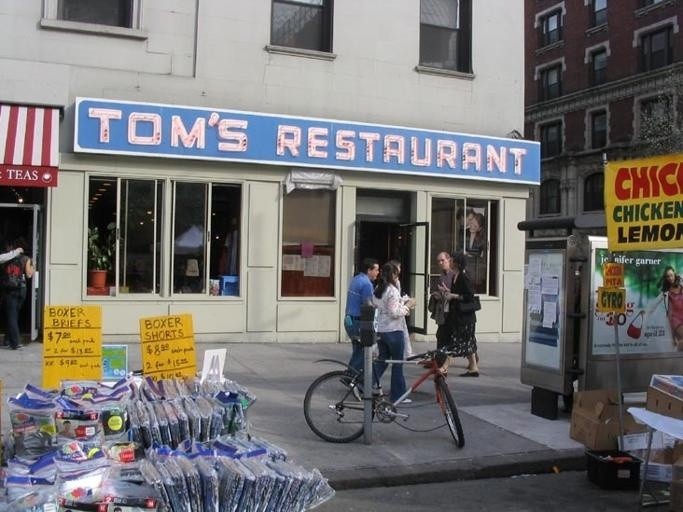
left=645, top=266, right=683, bottom=352
left=0, top=247, right=24, bottom=264
left=458, top=208, right=486, bottom=260
left=342, top=254, right=416, bottom=404
left=4, top=238, right=34, bottom=350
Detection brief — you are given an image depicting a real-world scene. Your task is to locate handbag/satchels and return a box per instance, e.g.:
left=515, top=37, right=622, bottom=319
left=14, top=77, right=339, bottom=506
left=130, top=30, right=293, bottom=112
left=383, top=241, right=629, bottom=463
left=458, top=296, right=480, bottom=313
left=627, top=324, right=641, bottom=338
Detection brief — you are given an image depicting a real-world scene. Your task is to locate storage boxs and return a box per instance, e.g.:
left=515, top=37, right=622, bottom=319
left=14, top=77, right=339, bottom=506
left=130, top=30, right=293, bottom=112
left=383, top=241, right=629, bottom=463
left=568, top=374, right=683, bottom=512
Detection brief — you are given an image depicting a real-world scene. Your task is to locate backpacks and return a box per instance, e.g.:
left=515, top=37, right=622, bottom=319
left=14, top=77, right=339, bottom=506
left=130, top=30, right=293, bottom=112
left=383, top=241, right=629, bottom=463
left=2, top=257, right=23, bottom=289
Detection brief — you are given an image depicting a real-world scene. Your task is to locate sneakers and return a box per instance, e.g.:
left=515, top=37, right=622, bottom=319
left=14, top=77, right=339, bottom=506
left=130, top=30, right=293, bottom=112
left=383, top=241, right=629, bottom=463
left=340, top=377, right=379, bottom=401
left=460, top=356, right=480, bottom=377
left=392, top=397, right=412, bottom=403
left=0, top=343, right=24, bottom=350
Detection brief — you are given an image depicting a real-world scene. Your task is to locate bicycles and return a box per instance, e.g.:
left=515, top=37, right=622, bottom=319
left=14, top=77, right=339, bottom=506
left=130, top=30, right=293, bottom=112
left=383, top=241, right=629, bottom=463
left=304, top=342, right=465, bottom=448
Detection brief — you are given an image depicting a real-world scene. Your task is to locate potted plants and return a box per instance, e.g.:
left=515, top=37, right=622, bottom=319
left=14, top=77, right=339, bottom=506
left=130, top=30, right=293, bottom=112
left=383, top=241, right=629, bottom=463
left=86, top=222, right=117, bottom=287
